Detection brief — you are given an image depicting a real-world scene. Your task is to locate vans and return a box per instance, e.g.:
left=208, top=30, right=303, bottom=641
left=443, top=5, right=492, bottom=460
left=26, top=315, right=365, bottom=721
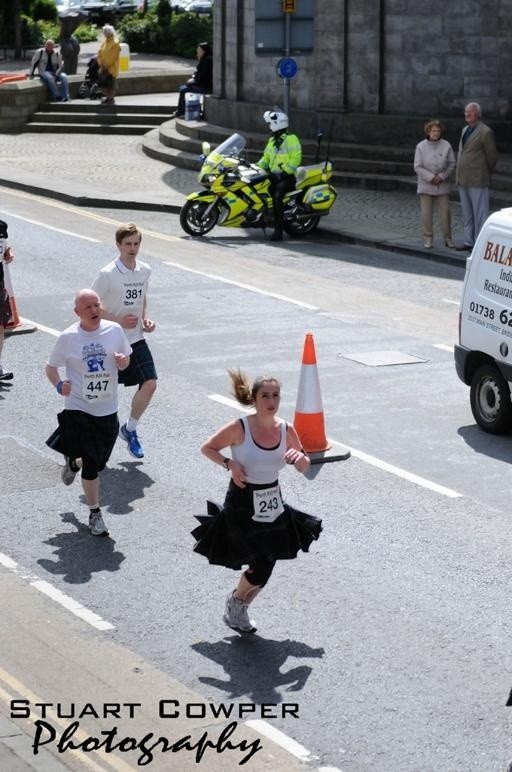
left=454, top=206, right=511, bottom=436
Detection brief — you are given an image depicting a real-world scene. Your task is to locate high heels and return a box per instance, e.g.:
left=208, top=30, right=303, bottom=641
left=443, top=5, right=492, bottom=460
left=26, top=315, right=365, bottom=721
left=424, top=240, right=431, bottom=248
left=445, top=240, right=455, bottom=248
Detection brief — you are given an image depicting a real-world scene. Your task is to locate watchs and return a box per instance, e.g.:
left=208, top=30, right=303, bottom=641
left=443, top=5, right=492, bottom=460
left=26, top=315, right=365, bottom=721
left=222, top=458, right=230, bottom=472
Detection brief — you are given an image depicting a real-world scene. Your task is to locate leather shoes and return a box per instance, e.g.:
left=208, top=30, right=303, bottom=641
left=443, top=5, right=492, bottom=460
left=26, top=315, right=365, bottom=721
left=456, top=244, right=473, bottom=251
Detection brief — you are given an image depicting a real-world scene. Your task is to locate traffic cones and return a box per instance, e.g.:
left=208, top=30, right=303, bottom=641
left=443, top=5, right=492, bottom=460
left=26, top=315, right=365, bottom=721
left=3, top=242, right=40, bottom=337
left=289, top=329, right=351, bottom=470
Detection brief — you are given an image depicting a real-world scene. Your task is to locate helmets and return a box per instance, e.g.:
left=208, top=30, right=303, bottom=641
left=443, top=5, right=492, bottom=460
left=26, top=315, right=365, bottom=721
left=263, top=108, right=290, bottom=133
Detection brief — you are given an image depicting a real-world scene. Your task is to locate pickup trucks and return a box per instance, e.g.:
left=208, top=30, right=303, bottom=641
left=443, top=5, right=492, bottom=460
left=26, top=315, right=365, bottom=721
left=81, top=0, right=138, bottom=17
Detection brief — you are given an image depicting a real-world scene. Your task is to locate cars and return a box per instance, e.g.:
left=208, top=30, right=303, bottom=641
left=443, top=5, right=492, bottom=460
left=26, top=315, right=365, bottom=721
left=54, top=0, right=104, bottom=16
left=170, top=0, right=213, bottom=17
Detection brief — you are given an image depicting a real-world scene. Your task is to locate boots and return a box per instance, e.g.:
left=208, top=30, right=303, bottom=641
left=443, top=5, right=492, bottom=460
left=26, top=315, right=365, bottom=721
left=270, top=213, right=283, bottom=241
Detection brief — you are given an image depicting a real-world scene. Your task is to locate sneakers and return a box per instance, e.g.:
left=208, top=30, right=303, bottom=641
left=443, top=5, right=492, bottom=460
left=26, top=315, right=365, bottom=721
left=60, top=453, right=77, bottom=486
left=119, top=422, right=144, bottom=458
left=0, top=366, right=13, bottom=380
left=89, top=511, right=110, bottom=535
left=223, top=588, right=253, bottom=632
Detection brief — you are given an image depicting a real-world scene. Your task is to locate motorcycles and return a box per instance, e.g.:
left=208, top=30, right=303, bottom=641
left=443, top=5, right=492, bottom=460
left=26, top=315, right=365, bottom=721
left=178, top=117, right=341, bottom=241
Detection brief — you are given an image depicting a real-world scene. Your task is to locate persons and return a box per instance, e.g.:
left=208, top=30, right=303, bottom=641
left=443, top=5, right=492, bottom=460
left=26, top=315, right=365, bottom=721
left=97, top=23, right=121, bottom=104
left=0, top=220, right=14, bottom=381
left=90, top=223, right=158, bottom=459
left=190, top=370, right=322, bottom=633
left=45, top=289, right=134, bottom=537
left=413, top=119, right=457, bottom=248
left=255, top=110, right=302, bottom=241
left=30, top=40, right=69, bottom=101
left=172, top=42, right=213, bottom=117
left=456, top=103, right=498, bottom=251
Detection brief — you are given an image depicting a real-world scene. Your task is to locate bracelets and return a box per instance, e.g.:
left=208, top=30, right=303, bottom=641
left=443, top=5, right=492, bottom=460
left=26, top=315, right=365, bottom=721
left=57, top=381, right=63, bottom=393
